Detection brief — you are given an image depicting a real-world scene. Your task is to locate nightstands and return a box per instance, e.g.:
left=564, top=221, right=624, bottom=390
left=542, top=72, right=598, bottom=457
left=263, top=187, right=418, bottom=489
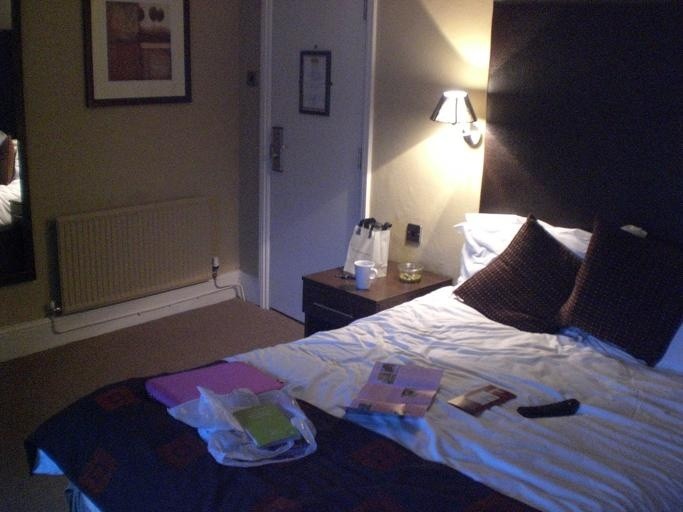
left=302, top=259, right=453, bottom=338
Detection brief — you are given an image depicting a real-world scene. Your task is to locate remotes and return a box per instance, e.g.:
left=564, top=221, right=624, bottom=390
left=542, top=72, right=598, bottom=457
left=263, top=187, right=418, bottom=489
left=518, top=397, right=580, bottom=418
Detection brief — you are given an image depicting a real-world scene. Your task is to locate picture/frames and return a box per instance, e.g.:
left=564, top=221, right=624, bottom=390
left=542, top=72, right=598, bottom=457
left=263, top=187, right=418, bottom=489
left=81, top=0, right=193, bottom=109
left=298, top=49, right=333, bottom=117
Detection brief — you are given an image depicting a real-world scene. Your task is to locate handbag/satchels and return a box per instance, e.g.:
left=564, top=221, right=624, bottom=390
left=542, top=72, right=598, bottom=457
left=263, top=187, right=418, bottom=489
left=343, top=217, right=392, bottom=279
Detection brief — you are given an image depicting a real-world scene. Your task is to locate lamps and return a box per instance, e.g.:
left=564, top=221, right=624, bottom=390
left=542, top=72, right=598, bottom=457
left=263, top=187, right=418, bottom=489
left=431, top=90, right=484, bottom=150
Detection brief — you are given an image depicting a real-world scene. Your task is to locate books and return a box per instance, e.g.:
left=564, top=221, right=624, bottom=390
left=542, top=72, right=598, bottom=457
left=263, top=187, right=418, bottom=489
left=233, top=404, right=302, bottom=446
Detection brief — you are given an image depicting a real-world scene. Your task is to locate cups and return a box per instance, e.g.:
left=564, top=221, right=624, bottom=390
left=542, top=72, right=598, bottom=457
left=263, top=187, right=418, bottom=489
left=354, top=260, right=379, bottom=290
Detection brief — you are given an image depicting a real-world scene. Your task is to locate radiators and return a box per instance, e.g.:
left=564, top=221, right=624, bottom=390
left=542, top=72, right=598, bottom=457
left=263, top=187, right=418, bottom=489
left=54, top=194, right=212, bottom=316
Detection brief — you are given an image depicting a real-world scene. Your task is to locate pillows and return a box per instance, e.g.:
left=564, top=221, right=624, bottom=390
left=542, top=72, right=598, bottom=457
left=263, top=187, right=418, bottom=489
left=450, top=211, right=683, bottom=369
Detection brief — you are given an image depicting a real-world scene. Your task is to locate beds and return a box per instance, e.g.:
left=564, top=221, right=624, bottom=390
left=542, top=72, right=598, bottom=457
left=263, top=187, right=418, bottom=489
left=27, top=0, right=683, bottom=511
